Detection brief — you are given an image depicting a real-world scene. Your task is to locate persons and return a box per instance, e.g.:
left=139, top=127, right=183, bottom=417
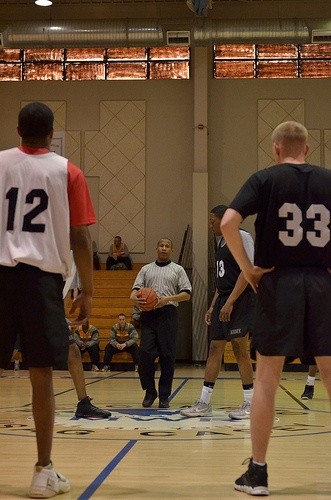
left=90, top=240, right=101, bottom=271
left=129, top=236, right=193, bottom=408
left=179, top=204, right=256, bottom=419
left=9, top=288, right=140, bottom=372
left=284, top=356, right=318, bottom=400
left=105, top=236, right=133, bottom=270
left=219, top=121, right=331, bottom=496
left=0, top=100, right=98, bottom=499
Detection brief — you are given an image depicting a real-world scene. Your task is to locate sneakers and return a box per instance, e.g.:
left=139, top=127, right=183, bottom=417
left=29, top=459, right=71, bottom=498
left=159, top=397, right=169, bottom=408
left=75, top=395, right=111, bottom=418
left=301, top=384, right=315, bottom=399
left=142, top=389, right=157, bottom=407
left=228, top=400, right=251, bottom=419
left=180, top=400, right=212, bottom=417
left=233, top=456, right=269, bottom=495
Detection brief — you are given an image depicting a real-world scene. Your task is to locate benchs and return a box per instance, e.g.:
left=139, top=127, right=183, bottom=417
left=64, top=262, right=152, bottom=363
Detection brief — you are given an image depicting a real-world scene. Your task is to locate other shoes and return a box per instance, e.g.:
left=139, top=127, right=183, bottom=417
left=92, top=364, right=100, bottom=371
left=102, top=365, right=109, bottom=372
left=135, top=365, right=138, bottom=372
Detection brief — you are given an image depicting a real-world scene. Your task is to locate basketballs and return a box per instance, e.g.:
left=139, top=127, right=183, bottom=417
left=137, top=288, right=157, bottom=311
